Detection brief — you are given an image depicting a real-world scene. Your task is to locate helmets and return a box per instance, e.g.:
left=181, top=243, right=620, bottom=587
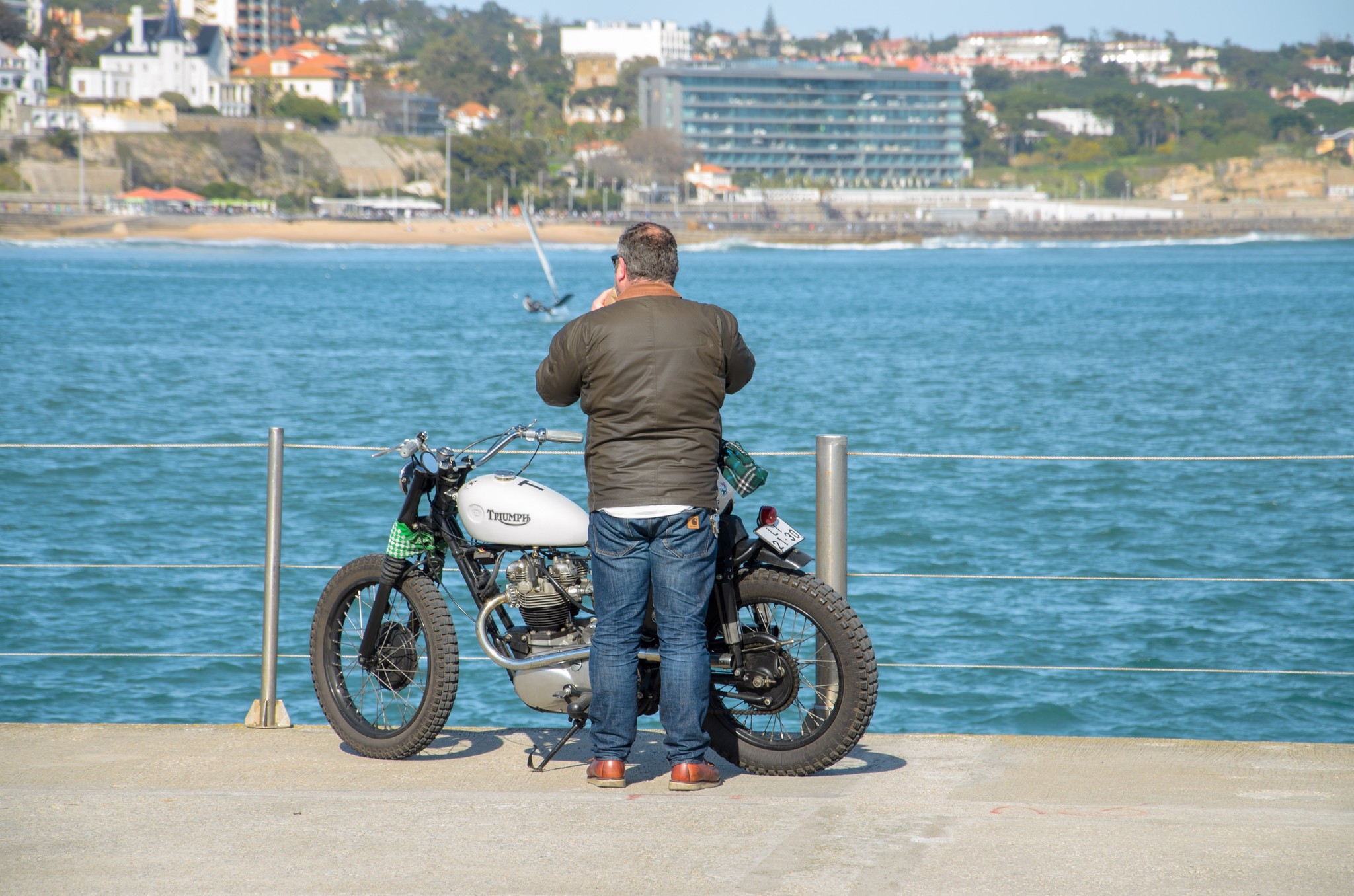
left=717, top=467, right=734, bottom=516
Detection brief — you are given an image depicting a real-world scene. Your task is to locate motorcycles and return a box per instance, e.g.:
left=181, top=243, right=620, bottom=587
left=308, top=417, right=880, bottom=780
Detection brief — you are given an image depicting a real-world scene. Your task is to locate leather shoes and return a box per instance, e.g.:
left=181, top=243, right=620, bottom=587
left=669, top=758, right=721, bottom=789
left=588, top=757, right=626, bottom=786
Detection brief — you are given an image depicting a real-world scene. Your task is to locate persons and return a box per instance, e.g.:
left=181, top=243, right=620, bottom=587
left=534, top=220, right=755, bottom=791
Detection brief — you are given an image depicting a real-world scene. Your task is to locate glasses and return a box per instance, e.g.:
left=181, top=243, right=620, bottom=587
left=611, top=255, right=621, bottom=266
